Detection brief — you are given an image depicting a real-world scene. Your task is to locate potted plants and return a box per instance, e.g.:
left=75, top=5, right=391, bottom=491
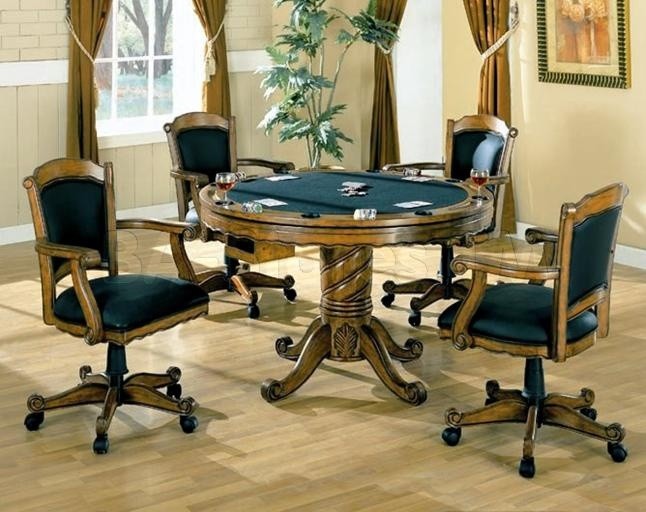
left=253, top=0, right=400, bottom=172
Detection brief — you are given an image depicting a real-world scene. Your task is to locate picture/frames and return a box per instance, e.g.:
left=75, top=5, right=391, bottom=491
left=536, top=0, right=631, bottom=89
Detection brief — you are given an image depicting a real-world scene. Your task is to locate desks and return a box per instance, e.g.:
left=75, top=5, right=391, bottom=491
left=198, top=172, right=495, bottom=407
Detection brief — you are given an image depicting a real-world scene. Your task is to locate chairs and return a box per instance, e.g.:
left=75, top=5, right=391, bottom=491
left=164, top=112, right=297, bottom=319
left=381, top=114, right=519, bottom=327
left=24, top=159, right=210, bottom=456
left=438, top=181, right=629, bottom=479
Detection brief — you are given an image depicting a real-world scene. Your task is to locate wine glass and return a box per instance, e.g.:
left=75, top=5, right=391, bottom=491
left=470, top=168, right=490, bottom=200
left=214, top=173, right=237, bottom=206
